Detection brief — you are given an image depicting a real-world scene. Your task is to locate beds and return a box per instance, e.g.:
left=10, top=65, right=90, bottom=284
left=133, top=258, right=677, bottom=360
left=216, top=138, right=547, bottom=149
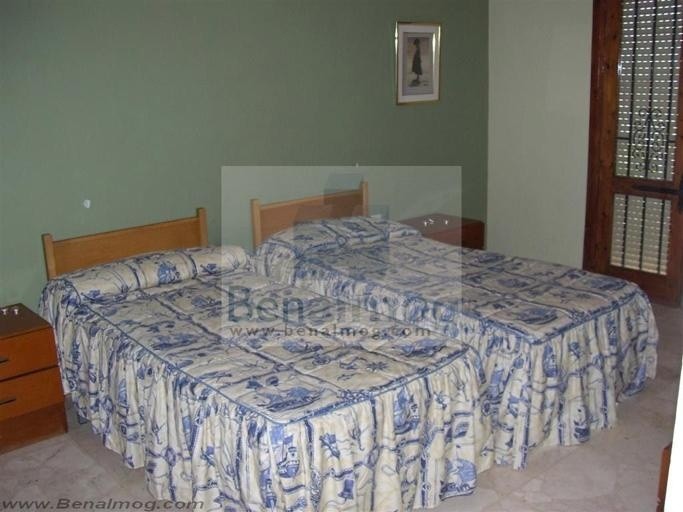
left=34, top=206, right=488, bottom=512
left=247, top=182, right=660, bottom=470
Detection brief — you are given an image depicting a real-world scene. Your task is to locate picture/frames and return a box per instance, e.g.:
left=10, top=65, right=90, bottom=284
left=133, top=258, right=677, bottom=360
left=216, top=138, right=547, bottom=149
left=395, top=21, right=440, bottom=106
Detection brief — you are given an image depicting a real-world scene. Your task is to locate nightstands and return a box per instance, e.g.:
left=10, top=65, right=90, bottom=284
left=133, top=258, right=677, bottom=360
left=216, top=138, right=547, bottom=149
left=0, top=303, right=68, bottom=455
left=397, top=213, right=484, bottom=250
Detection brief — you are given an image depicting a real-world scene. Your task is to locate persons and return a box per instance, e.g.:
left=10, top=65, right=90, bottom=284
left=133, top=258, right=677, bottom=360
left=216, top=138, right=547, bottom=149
left=410, top=37, right=423, bottom=85
